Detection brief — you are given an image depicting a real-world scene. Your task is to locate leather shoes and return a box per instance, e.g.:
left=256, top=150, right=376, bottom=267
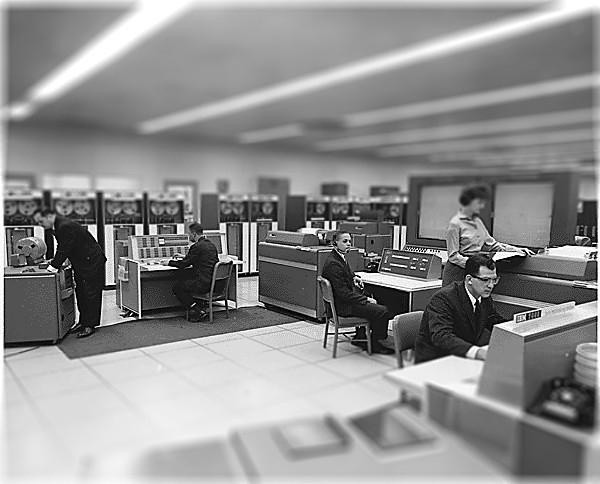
left=76, top=326, right=96, bottom=338
left=350, top=336, right=367, bottom=347
left=188, top=310, right=210, bottom=322
left=361, top=341, right=396, bottom=355
left=68, top=322, right=84, bottom=334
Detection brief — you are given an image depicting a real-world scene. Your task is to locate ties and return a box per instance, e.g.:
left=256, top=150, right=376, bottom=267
left=474, top=301, right=483, bottom=330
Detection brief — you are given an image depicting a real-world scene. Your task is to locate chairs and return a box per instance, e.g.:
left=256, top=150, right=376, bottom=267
left=393, top=311, right=426, bottom=403
left=185, top=261, right=234, bottom=323
left=316, top=274, right=372, bottom=359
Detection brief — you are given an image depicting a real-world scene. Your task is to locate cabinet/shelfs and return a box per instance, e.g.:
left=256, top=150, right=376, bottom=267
left=4, top=264, right=74, bottom=348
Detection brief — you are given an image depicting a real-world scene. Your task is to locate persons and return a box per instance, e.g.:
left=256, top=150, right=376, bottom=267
left=321, top=231, right=395, bottom=354
left=442, top=187, right=536, bottom=288
left=160, top=222, right=224, bottom=322
left=32, top=209, right=107, bottom=338
left=415, top=253, right=509, bottom=364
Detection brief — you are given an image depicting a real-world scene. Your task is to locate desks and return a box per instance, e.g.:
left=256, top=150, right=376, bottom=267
left=118, top=252, right=246, bottom=320
left=354, top=270, right=443, bottom=311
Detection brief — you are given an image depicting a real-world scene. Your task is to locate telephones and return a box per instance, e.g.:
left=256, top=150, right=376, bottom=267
left=362, top=261, right=379, bottom=273
left=525, top=376, right=596, bottom=428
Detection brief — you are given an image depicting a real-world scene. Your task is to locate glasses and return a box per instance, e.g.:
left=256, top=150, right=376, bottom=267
left=467, top=272, right=502, bottom=284
